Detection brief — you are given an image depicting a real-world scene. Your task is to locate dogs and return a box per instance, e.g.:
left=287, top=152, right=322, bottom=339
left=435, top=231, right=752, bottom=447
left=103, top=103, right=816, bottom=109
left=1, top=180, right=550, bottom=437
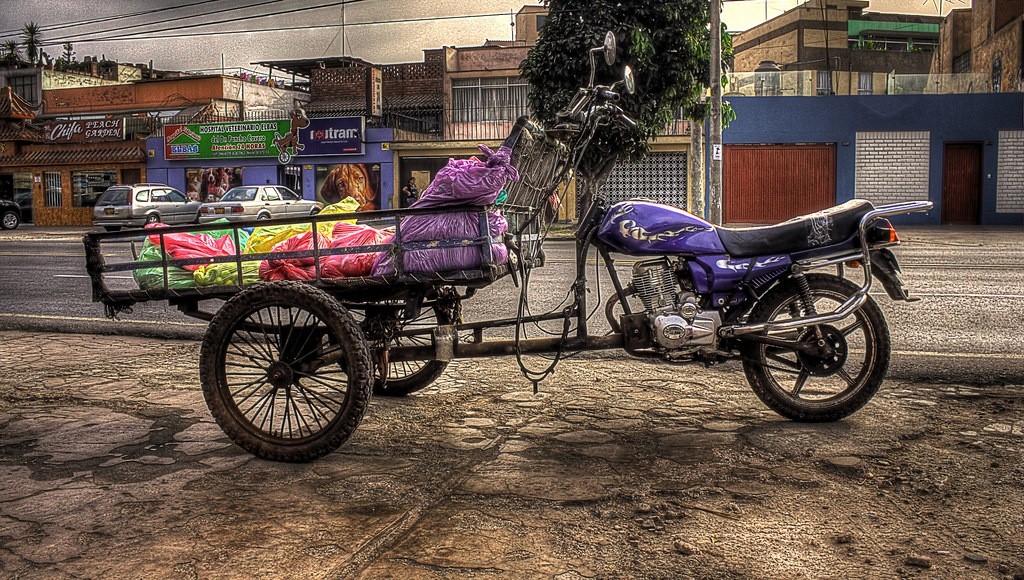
left=320, top=164, right=378, bottom=212
left=186, top=167, right=232, bottom=203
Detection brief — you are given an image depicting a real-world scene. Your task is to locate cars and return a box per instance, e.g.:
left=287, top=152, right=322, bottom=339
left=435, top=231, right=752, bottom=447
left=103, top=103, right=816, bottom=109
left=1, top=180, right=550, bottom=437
left=0, top=200, right=22, bottom=231
left=198, top=185, right=324, bottom=223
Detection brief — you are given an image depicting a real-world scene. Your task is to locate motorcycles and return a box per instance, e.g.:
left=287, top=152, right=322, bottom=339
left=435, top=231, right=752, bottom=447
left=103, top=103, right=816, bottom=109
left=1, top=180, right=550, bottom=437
left=82, top=29, right=934, bottom=465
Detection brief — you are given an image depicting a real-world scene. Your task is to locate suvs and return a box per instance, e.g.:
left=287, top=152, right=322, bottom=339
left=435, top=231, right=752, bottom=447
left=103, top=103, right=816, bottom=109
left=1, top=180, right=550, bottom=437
left=91, top=182, right=204, bottom=232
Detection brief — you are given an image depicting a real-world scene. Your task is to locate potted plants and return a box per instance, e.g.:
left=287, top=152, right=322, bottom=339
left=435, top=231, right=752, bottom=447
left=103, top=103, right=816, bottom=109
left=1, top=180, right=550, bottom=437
left=20, top=22, right=43, bottom=68
left=43, top=53, right=54, bottom=69
left=3, top=40, right=22, bottom=69
